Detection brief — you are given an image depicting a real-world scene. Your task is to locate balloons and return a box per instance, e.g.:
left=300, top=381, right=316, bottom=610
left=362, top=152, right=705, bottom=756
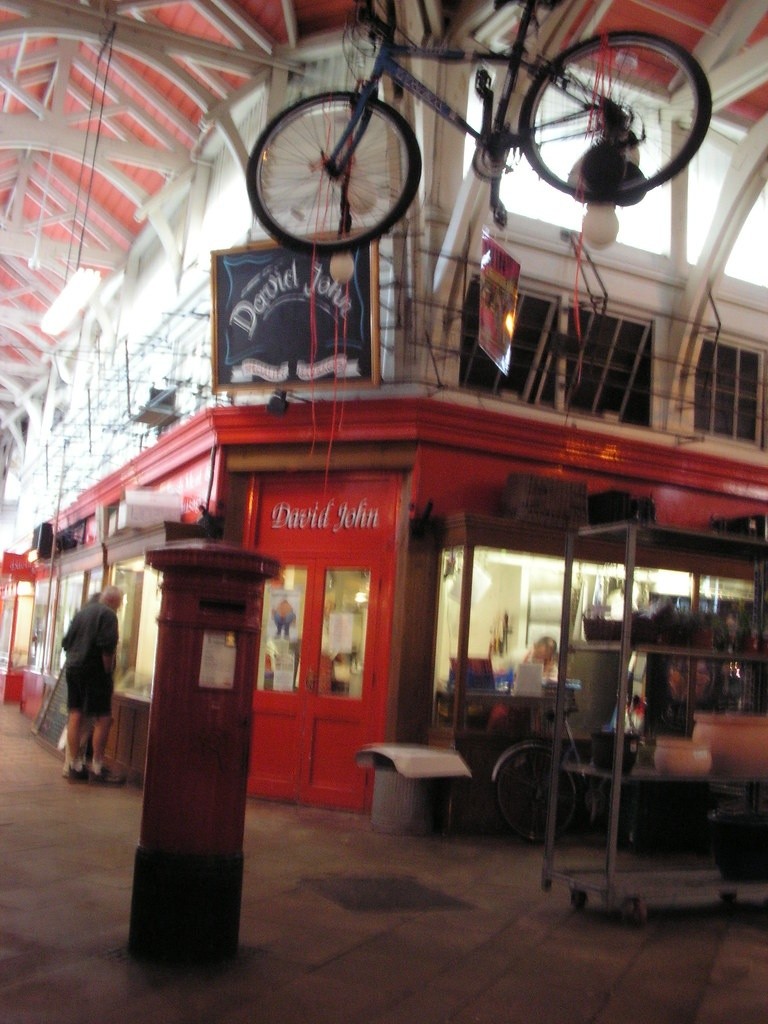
left=347, top=161, right=377, bottom=214
left=328, top=253, right=354, bottom=286
left=567, top=127, right=647, bottom=251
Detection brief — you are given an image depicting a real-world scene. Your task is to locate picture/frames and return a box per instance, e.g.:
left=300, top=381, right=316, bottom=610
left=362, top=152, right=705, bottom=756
left=209, top=226, right=380, bottom=395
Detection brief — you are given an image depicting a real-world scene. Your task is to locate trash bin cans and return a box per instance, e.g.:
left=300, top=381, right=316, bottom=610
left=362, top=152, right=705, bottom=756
left=369, top=753, right=430, bottom=836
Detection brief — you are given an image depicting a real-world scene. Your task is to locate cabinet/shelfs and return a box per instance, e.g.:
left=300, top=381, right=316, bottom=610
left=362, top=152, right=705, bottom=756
left=539, top=518, right=768, bottom=925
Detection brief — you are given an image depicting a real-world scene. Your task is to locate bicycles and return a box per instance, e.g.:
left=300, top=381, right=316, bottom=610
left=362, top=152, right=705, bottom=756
left=245, top=0, right=712, bottom=260
left=490, top=695, right=671, bottom=854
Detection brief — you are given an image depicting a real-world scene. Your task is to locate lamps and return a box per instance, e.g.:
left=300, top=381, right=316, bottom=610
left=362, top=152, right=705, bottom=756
left=39, top=265, right=102, bottom=337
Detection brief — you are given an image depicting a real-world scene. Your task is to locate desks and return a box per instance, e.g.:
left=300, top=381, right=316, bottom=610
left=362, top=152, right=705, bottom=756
left=436, top=684, right=555, bottom=747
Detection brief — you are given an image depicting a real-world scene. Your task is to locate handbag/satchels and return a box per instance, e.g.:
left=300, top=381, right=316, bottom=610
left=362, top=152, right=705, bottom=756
left=57, top=725, right=68, bottom=754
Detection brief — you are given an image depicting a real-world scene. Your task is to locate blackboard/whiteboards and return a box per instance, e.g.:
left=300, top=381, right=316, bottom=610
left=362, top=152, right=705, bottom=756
left=210, top=225, right=383, bottom=396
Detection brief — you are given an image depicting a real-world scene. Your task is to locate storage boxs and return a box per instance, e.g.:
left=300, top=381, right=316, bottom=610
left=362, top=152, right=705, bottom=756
left=118, top=487, right=182, bottom=529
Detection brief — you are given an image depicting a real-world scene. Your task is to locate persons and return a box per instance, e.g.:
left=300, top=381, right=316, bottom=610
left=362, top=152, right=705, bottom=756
left=61, top=586, right=126, bottom=787
left=493, top=637, right=558, bottom=678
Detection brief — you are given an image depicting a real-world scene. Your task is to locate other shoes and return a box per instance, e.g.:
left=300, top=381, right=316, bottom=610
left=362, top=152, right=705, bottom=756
left=67, top=764, right=89, bottom=784
left=88, top=766, right=126, bottom=787
